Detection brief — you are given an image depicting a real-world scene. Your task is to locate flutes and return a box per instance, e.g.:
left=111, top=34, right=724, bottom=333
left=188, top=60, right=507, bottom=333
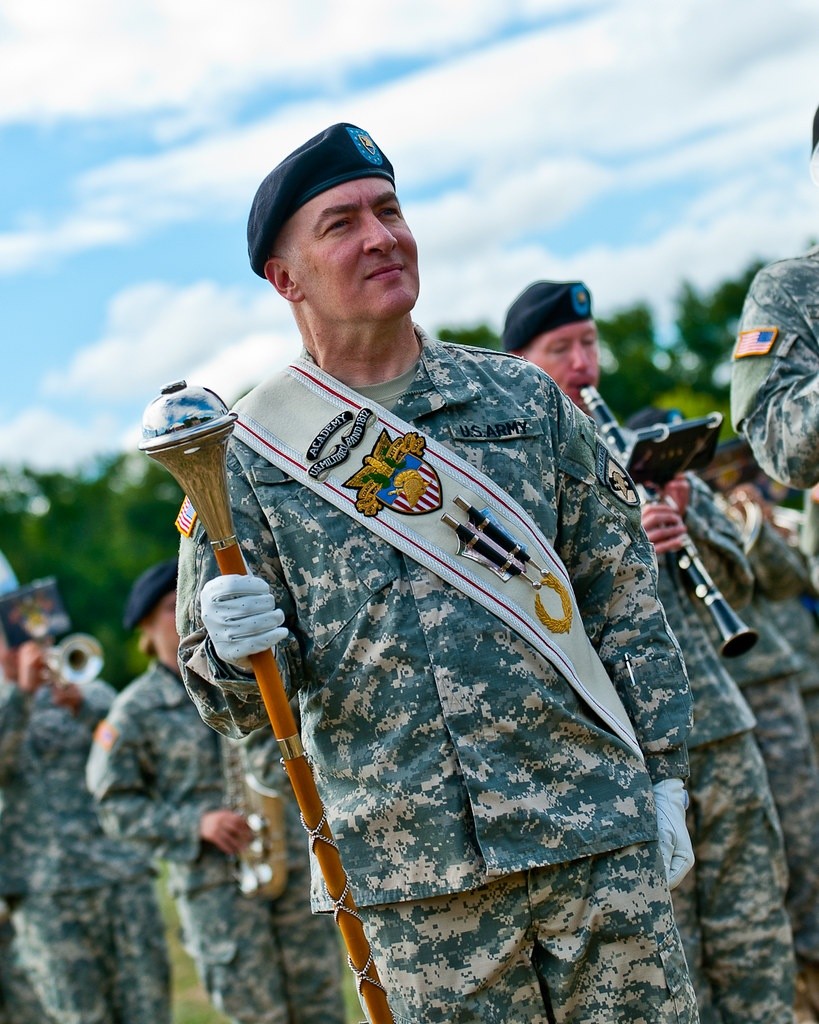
left=579, top=381, right=761, bottom=661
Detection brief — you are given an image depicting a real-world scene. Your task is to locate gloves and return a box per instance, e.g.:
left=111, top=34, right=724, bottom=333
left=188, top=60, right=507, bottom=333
left=652, top=778, right=695, bottom=891
left=200, top=542, right=289, bottom=672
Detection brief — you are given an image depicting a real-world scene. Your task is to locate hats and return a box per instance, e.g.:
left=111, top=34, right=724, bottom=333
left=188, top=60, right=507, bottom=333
left=501, top=280, right=594, bottom=353
left=248, top=123, right=396, bottom=281
left=121, top=560, right=178, bottom=631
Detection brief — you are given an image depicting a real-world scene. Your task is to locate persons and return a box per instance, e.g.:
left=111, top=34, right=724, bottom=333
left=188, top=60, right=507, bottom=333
left=0, top=554, right=347, bottom=1024
left=731, top=105, right=819, bottom=491
left=177, top=122, right=701, bottom=1024
left=502, top=284, right=819, bottom=1024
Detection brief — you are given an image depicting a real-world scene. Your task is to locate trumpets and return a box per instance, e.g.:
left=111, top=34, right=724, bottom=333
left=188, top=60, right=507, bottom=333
left=29, top=632, right=105, bottom=691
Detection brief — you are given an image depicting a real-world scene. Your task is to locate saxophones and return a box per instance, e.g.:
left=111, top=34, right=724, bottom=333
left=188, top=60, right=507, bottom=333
left=219, top=735, right=290, bottom=902
